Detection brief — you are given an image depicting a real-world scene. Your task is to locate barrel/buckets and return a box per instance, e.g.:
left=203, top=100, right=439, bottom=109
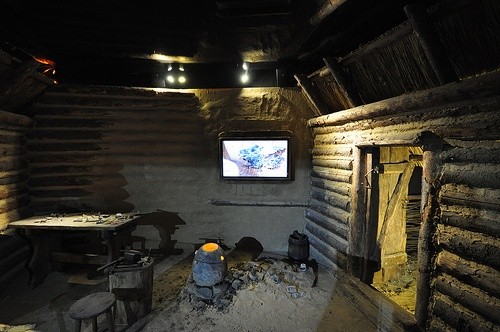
left=288, top=233, right=309, bottom=259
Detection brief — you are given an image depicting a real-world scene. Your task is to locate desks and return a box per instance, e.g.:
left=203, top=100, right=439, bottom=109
left=9, top=210, right=147, bottom=287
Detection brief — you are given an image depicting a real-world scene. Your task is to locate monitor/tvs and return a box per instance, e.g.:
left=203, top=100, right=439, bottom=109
left=218, top=137, right=291, bottom=182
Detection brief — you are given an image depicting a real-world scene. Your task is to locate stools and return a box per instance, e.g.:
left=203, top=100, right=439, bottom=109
left=70, top=292, right=116, bottom=332
